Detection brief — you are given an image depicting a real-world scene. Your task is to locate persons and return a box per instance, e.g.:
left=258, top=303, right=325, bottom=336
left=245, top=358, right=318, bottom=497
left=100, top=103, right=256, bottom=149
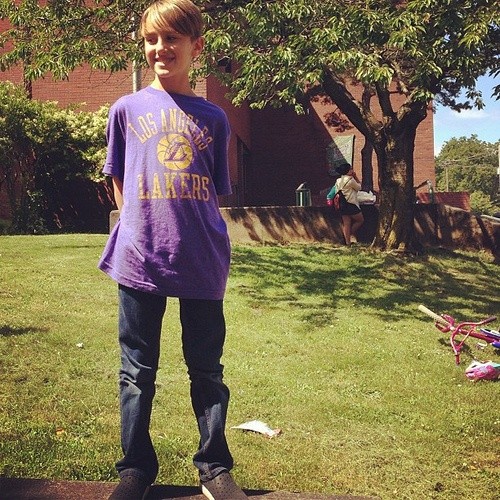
left=326, top=163, right=364, bottom=246
left=97, top=0, right=248, bottom=499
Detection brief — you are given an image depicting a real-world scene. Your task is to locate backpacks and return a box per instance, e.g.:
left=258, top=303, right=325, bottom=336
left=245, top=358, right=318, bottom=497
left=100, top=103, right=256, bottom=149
left=333, top=177, right=354, bottom=214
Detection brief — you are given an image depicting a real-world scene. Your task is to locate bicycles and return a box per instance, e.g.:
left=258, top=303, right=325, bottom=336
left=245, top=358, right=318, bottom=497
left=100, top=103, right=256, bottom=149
left=417, top=305, right=499, bottom=365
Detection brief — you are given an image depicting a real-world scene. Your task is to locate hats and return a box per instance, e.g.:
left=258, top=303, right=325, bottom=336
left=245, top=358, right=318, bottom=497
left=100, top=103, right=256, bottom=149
left=336, top=163, right=350, bottom=174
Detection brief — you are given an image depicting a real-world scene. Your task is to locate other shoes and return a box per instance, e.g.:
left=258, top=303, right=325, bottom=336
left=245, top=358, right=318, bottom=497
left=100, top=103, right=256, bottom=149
left=350, top=235, right=357, bottom=243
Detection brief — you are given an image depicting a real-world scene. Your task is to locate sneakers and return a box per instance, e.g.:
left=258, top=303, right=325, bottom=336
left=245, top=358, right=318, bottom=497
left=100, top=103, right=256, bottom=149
left=199, top=470, right=249, bottom=500
left=109, top=475, right=150, bottom=500
